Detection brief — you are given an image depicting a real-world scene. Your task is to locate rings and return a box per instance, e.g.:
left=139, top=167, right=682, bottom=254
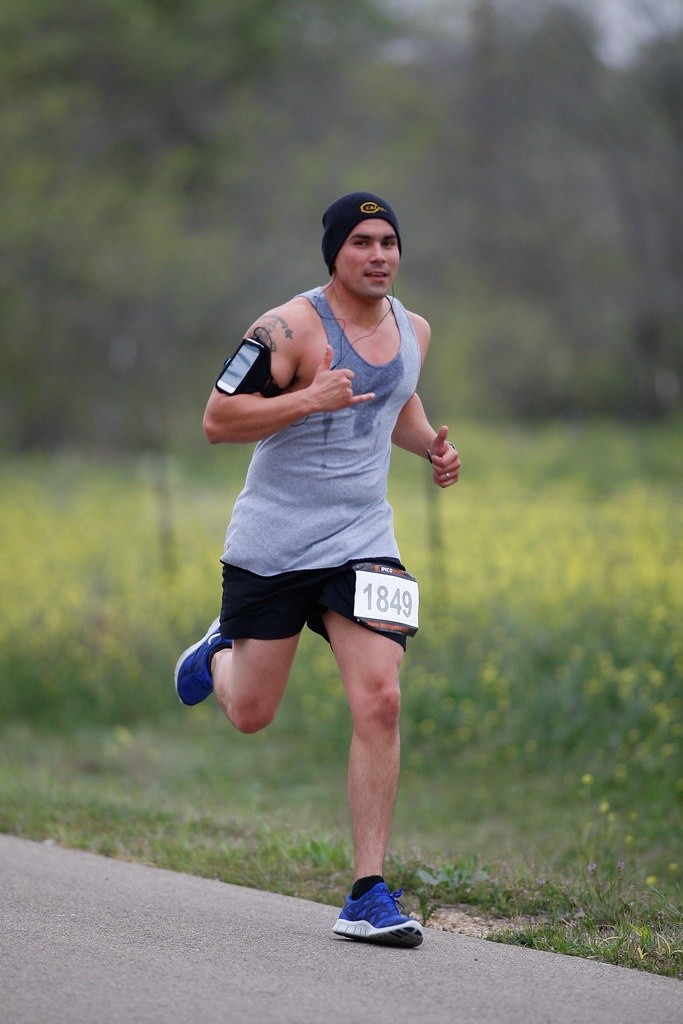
left=446, top=471, right=450, bottom=480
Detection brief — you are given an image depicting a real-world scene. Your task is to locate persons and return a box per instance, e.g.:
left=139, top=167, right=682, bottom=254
left=173, top=192, right=462, bottom=945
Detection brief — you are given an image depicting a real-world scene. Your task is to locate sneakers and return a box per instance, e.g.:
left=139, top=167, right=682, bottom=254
left=174, top=615, right=233, bottom=707
left=332, top=883, right=424, bottom=948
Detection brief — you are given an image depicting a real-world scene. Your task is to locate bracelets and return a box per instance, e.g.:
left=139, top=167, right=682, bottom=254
left=427, top=443, right=456, bottom=464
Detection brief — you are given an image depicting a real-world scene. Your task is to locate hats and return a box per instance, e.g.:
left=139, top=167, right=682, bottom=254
left=322, top=192, right=402, bottom=276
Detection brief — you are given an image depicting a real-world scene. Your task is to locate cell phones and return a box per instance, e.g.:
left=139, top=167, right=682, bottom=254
left=215, top=337, right=265, bottom=395
